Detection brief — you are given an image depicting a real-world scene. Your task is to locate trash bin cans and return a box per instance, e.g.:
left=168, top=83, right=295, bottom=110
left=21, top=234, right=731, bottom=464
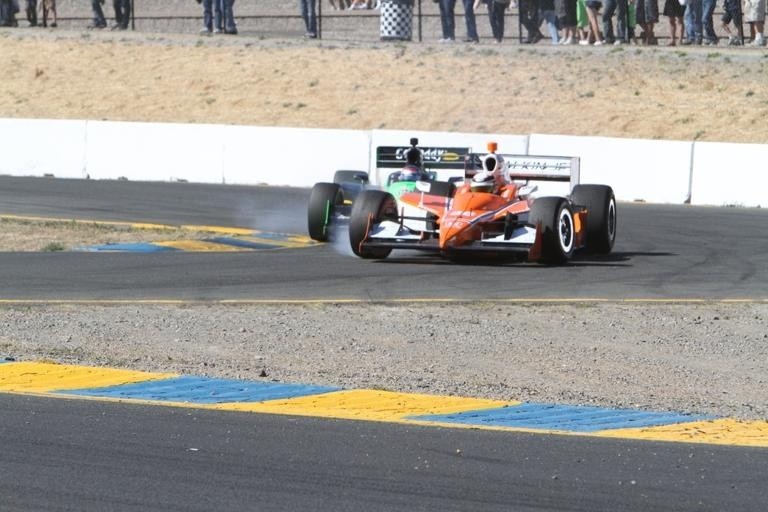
left=379, top=0, right=415, bottom=42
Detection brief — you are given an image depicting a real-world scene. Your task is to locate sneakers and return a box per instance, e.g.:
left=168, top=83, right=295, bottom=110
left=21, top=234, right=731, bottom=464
left=522, top=31, right=543, bottom=44
left=558, top=36, right=625, bottom=46
left=728, top=35, right=766, bottom=46
left=197, top=26, right=222, bottom=33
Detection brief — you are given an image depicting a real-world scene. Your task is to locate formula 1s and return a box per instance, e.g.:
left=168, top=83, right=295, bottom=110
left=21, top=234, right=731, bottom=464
left=307, top=139, right=616, bottom=264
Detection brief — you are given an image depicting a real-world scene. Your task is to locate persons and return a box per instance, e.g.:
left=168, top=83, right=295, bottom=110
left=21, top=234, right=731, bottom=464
left=113, top=0, right=130, bottom=29
left=301, top=0, right=317, bottom=37
left=196, top=1, right=221, bottom=33
left=220, top=1, right=238, bottom=33
left=328, top=0, right=381, bottom=10
left=0, top=0, right=57, bottom=26
left=88, top=0, right=107, bottom=28
left=434, top=0, right=768, bottom=49
left=399, top=164, right=421, bottom=181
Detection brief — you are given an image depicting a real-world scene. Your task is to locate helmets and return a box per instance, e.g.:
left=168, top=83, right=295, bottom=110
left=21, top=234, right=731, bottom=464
left=400, top=165, right=420, bottom=181
